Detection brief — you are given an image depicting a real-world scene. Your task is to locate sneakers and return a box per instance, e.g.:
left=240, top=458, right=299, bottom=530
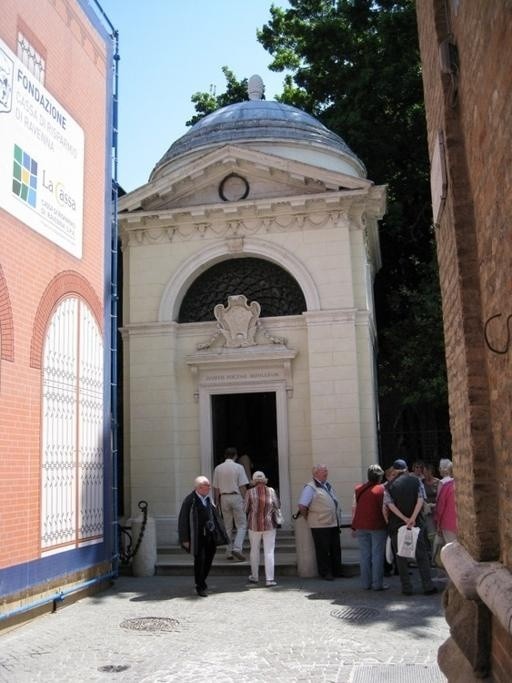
left=266, top=581, right=277, bottom=586
left=249, top=575, right=258, bottom=583
left=227, top=548, right=246, bottom=560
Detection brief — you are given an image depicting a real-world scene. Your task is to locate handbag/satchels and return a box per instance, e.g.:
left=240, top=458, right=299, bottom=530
left=397, top=526, right=420, bottom=558
left=274, top=509, right=284, bottom=525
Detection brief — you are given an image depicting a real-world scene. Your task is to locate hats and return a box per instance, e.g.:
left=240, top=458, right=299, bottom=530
left=253, top=471, right=265, bottom=479
left=394, top=459, right=407, bottom=469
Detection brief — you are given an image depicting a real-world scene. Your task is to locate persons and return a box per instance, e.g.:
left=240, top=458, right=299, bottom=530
left=212, top=447, right=248, bottom=561
left=242, top=470, right=283, bottom=587
left=350, top=457, right=457, bottom=596
left=297, top=464, right=352, bottom=580
left=238, top=448, right=252, bottom=480
left=177, top=476, right=231, bottom=597
